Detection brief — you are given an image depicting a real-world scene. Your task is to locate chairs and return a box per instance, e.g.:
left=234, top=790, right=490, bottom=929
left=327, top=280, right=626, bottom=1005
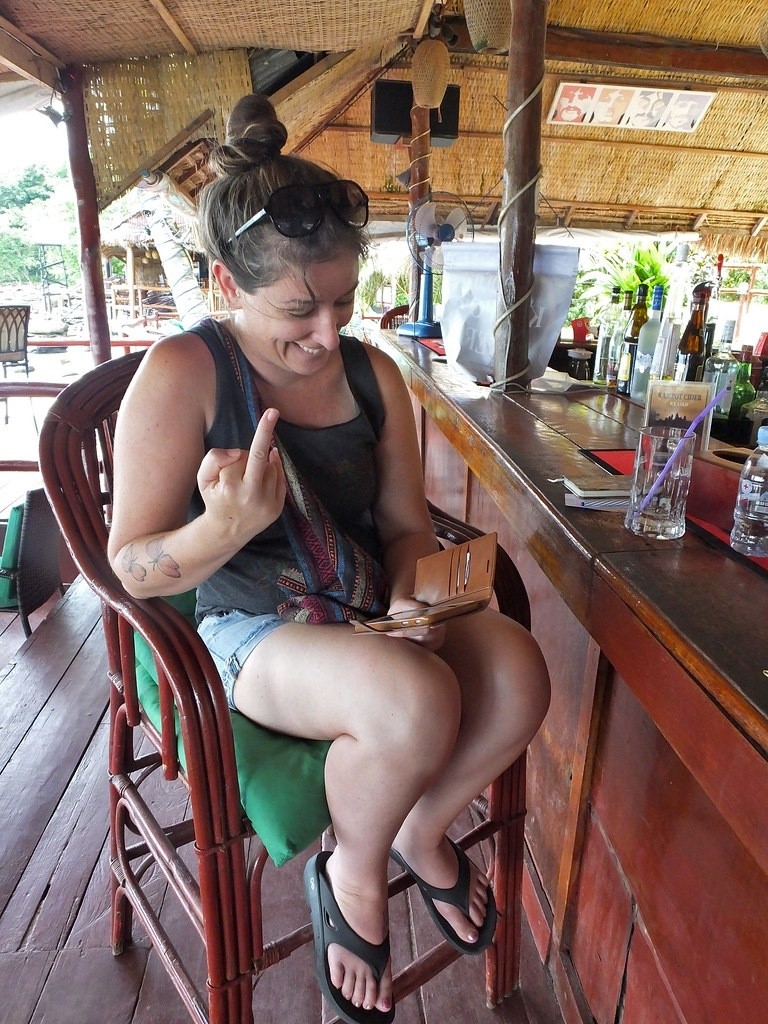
left=39, top=352, right=527, bottom=1024
left=379, top=306, right=409, bottom=330
left=0, top=490, right=66, bottom=638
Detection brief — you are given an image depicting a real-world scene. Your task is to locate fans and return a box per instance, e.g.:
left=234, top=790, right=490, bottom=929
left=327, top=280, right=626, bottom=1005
left=396, top=191, right=474, bottom=339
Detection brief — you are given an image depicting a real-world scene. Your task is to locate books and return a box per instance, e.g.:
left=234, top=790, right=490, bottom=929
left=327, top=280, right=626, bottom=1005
left=561, top=473, right=634, bottom=499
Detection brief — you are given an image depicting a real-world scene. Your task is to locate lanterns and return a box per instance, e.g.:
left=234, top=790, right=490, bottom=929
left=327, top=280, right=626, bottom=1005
left=463, top=0, right=512, bottom=55
left=411, top=40, right=450, bottom=109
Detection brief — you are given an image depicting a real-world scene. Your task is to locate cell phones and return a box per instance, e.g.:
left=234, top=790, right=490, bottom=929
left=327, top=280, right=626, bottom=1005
left=354, top=598, right=485, bottom=632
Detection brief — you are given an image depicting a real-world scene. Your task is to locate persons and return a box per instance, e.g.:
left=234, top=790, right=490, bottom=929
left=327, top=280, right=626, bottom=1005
left=107, top=95, right=553, bottom=1024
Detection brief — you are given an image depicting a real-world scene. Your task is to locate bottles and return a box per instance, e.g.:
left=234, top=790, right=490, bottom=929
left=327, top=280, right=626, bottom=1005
left=567, top=349, right=593, bottom=380
left=594, top=282, right=756, bottom=449
left=730, top=426, right=768, bottom=559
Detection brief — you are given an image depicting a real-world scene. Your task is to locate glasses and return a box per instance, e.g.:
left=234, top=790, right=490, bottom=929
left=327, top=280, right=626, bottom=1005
left=224, top=180, right=368, bottom=244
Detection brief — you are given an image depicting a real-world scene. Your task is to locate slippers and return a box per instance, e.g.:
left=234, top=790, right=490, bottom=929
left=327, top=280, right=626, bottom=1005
left=390, top=832, right=498, bottom=956
left=303, top=850, right=395, bottom=1024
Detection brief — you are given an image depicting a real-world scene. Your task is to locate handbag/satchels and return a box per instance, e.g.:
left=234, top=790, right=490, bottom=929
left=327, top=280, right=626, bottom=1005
left=440, top=240, right=580, bottom=386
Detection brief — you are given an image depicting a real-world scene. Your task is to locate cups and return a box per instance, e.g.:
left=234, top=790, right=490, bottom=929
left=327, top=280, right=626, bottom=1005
left=623, top=427, right=695, bottom=541
